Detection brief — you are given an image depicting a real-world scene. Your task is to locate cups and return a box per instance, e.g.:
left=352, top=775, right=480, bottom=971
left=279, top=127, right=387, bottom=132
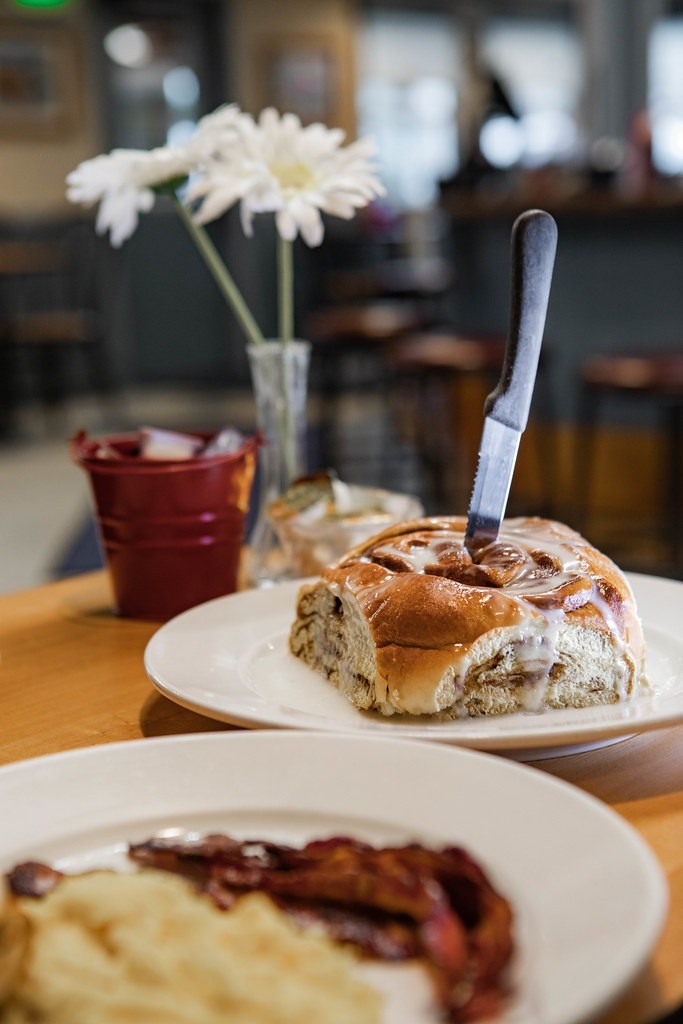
left=270, top=479, right=423, bottom=588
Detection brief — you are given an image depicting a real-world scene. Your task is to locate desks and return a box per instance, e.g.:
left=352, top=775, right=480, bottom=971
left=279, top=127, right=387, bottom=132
left=0, top=543, right=683, bottom=1024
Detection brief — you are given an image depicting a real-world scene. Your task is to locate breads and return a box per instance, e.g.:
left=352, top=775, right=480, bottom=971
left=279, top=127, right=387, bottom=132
left=289, top=514, right=642, bottom=715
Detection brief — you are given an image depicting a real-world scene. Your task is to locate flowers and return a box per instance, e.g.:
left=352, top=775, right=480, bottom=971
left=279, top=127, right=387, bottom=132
left=66, top=109, right=392, bottom=485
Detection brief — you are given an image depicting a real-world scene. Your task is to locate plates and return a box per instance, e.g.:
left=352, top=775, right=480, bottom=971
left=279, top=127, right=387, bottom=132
left=0, top=731, right=669, bottom=1024
left=142, top=573, right=683, bottom=763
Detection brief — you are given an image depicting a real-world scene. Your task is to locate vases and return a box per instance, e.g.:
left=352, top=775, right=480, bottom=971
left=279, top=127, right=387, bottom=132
left=241, top=341, right=315, bottom=587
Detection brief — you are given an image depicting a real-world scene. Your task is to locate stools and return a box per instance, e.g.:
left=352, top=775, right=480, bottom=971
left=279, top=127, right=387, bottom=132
left=314, top=300, right=683, bottom=569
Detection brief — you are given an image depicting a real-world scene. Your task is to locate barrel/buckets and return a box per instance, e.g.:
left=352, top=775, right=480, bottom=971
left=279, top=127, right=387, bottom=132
left=61, top=429, right=268, bottom=622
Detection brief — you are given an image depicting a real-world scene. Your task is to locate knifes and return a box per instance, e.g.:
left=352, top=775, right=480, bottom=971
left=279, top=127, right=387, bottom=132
left=461, top=211, right=558, bottom=565
